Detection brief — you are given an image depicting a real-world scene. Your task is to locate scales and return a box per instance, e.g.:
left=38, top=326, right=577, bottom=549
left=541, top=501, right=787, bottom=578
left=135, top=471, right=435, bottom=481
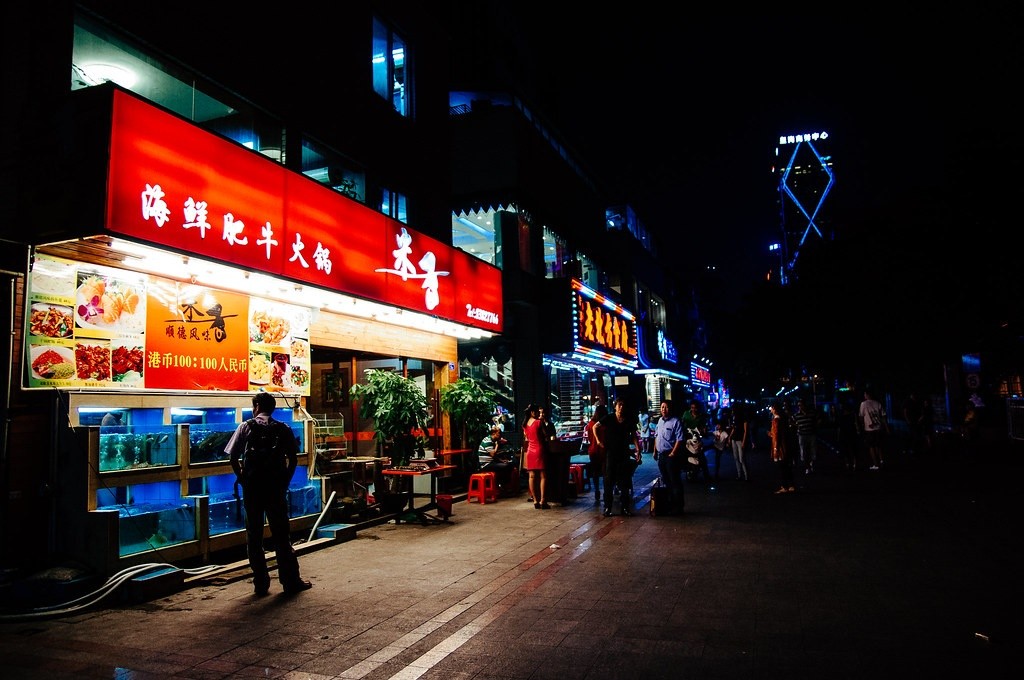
left=409, top=457, right=440, bottom=469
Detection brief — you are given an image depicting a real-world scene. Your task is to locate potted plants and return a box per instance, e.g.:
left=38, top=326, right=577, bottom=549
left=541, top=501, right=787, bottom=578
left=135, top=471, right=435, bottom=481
left=439, top=378, right=499, bottom=491
left=348, top=368, right=432, bottom=511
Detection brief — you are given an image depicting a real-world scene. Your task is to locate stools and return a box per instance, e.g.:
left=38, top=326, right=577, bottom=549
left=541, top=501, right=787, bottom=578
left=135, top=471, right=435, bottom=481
left=570, top=464, right=592, bottom=494
left=504, top=467, right=520, bottom=498
left=468, top=472, right=501, bottom=504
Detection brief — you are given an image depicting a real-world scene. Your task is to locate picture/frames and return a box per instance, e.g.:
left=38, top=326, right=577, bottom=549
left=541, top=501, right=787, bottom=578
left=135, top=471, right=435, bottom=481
left=322, top=368, right=349, bottom=408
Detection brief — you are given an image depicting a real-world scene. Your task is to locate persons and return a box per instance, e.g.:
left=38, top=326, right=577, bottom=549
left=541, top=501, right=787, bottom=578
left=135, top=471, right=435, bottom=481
left=585, top=406, right=610, bottom=499
left=101, top=411, right=127, bottom=427
left=859, top=390, right=889, bottom=472
left=223, top=392, right=313, bottom=594
left=592, top=399, right=640, bottom=517
left=526, top=406, right=556, bottom=503
left=478, top=427, right=511, bottom=496
left=899, top=391, right=935, bottom=454
left=765, top=401, right=796, bottom=494
left=788, top=400, right=820, bottom=476
left=636, top=400, right=767, bottom=510
left=522, top=404, right=551, bottom=509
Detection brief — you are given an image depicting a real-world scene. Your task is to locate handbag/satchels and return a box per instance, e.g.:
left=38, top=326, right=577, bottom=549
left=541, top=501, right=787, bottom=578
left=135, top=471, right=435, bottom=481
left=579, top=443, right=590, bottom=455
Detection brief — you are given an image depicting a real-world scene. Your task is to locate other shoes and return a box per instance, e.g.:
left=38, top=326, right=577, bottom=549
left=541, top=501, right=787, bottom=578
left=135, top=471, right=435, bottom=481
left=880, top=460, right=883, bottom=463
left=287, top=581, right=312, bottom=599
left=735, top=477, right=741, bottom=480
left=527, top=498, right=533, bottom=502
left=614, top=486, right=619, bottom=495
left=642, top=450, right=645, bottom=453
left=595, top=491, right=600, bottom=500
left=542, top=504, right=550, bottom=508
left=622, top=509, right=628, bottom=516
left=646, top=450, right=648, bottom=453
left=810, top=461, right=816, bottom=472
left=806, top=468, right=810, bottom=474
left=534, top=501, right=540, bottom=509
left=256, top=590, right=267, bottom=596
left=603, top=508, right=611, bottom=516
left=870, top=465, right=879, bottom=469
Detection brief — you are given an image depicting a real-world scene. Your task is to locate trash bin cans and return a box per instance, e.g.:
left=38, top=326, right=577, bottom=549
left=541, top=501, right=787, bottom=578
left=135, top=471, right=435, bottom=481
left=435, top=495, right=452, bottom=517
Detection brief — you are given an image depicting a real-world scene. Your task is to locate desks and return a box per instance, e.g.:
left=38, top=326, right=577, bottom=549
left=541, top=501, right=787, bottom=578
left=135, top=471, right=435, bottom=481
left=382, top=465, right=457, bottom=526
left=330, top=458, right=392, bottom=498
left=570, top=454, right=591, bottom=488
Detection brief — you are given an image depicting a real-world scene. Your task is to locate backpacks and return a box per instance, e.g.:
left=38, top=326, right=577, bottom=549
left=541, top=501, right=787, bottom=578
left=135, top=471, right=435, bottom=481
left=233, top=420, right=295, bottom=521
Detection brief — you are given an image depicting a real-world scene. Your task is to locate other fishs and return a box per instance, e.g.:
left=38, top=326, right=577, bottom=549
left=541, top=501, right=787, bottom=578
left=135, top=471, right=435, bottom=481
left=190, top=430, right=235, bottom=461
left=132, top=502, right=192, bottom=543
left=145, top=434, right=169, bottom=446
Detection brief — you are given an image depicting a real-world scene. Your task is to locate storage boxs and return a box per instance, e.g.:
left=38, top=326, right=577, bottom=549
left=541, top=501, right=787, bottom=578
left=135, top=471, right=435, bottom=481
left=98, top=421, right=322, bottom=557
